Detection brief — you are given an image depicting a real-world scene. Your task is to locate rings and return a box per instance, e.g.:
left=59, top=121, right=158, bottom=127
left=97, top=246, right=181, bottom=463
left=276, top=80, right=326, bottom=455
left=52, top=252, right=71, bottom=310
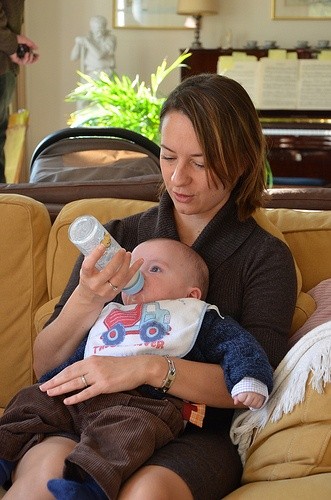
left=107, top=281, right=119, bottom=293
left=82, top=375, right=90, bottom=386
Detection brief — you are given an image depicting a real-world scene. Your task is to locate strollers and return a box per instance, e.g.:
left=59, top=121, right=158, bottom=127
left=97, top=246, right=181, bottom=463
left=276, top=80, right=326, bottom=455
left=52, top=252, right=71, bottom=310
left=29, top=127, right=161, bottom=182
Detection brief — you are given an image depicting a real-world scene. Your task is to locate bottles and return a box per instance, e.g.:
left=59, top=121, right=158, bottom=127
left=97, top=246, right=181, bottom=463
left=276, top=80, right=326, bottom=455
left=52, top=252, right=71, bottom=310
left=67, top=214, right=144, bottom=296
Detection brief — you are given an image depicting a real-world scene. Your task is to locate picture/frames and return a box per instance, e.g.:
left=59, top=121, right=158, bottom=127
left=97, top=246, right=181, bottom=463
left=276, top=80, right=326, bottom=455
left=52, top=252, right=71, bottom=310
left=112, top=0, right=197, bottom=31
left=270, top=0, right=331, bottom=21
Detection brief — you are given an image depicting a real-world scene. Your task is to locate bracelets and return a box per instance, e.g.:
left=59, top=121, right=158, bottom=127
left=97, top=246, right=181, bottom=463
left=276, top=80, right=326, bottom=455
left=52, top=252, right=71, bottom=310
left=156, top=354, right=176, bottom=394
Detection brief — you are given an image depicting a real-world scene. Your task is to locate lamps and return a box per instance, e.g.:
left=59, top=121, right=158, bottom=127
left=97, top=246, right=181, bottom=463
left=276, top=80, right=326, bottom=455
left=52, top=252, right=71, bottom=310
left=175, top=0, right=219, bottom=49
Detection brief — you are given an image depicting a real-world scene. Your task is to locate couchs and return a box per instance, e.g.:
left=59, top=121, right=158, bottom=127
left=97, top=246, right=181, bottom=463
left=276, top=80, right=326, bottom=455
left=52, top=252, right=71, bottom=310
left=0, top=193, right=331, bottom=500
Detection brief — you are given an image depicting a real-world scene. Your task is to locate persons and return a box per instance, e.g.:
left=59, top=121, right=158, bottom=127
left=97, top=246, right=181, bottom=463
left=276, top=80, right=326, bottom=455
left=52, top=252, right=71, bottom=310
left=70, top=14, right=115, bottom=111
left=0, top=239, right=275, bottom=500
left=0, top=72, right=296, bottom=500
left=0, top=0, right=40, bottom=184
